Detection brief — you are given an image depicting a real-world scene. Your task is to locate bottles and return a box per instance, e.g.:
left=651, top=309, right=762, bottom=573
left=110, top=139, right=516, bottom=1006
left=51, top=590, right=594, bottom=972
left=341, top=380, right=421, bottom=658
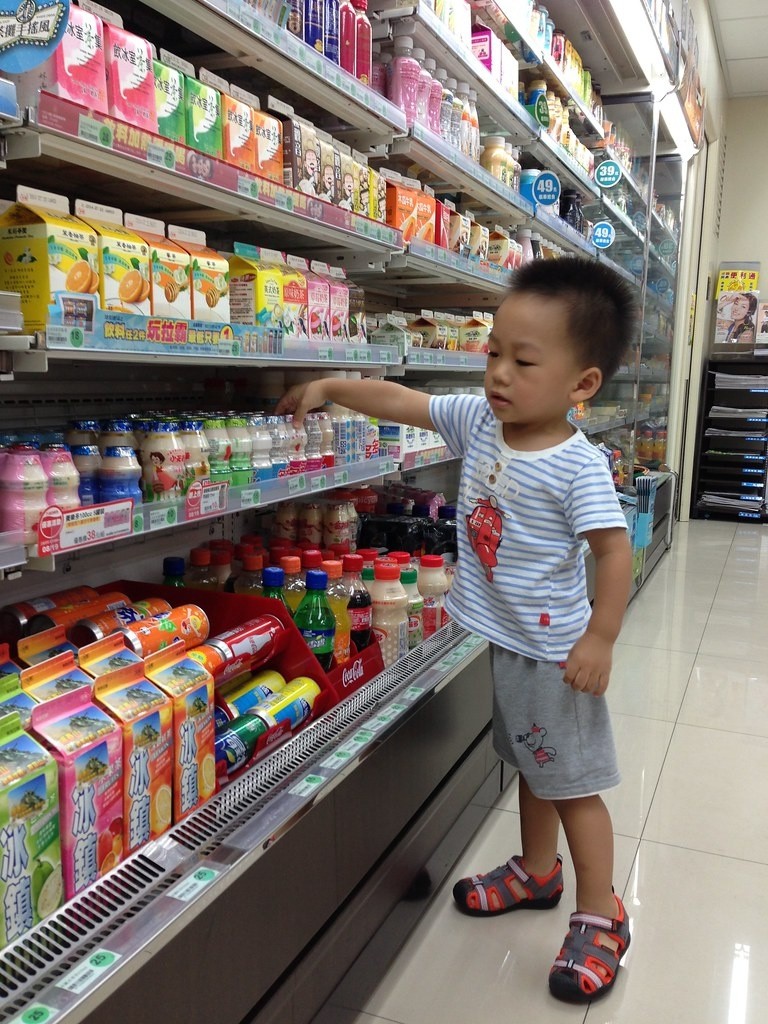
left=634, top=428, right=667, bottom=465
left=612, top=448, right=624, bottom=486
left=299, top=0, right=586, bottom=270
left=0, top=370, right=487, bottom=679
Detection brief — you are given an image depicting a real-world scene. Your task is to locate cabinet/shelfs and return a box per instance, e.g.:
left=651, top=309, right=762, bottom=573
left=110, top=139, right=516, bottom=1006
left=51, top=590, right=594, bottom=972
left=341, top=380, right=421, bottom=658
left=0, top=0, right=687, bottom=958
left=692, top=358, right=768, bottom=523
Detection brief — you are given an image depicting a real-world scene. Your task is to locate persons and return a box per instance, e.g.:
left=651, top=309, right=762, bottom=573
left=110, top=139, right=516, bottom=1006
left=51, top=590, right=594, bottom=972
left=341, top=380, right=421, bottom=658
left=716, top=293, right=768, bottom=343
left=272, top=258, right=640, bottom=1005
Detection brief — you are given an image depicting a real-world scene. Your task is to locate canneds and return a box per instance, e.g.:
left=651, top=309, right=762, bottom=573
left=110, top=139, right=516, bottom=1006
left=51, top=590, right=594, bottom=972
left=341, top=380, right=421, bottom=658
left=0, top=585, right=321, bottom=775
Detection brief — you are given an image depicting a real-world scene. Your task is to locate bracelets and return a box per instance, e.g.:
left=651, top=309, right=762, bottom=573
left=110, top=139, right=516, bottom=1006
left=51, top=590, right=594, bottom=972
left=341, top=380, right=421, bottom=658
left=717, top=308, right=722, bottom=314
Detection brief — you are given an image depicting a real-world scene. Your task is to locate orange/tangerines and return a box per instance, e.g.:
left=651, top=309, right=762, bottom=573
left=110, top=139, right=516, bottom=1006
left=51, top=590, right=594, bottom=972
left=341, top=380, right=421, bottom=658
left=66, top=260, right=151, bottom=303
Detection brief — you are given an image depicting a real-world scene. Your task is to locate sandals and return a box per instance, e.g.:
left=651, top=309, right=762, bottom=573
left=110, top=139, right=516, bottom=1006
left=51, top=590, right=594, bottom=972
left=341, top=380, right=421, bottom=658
left=549, top=884, right=631, bottom=1004
left=452, top=852, right=563, bottom=917
